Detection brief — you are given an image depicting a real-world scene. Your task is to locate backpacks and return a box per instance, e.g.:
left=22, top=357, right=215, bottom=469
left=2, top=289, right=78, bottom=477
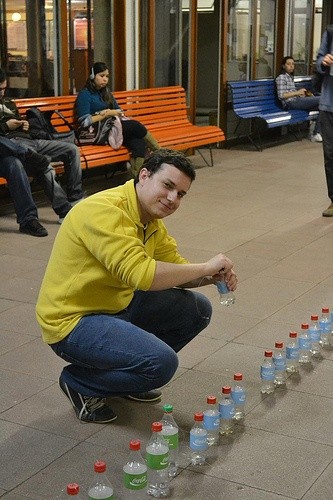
left=25, top=108, right=78, bottom=144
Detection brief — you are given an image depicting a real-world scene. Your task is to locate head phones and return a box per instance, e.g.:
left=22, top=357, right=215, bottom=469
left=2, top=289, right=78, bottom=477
left=89, top=66, right=94, bottom=80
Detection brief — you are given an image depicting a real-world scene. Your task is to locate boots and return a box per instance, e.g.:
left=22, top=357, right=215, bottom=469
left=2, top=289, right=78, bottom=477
left=129, top=157, right=144, bottom=179
left=143, top=133, right=161, bottom=152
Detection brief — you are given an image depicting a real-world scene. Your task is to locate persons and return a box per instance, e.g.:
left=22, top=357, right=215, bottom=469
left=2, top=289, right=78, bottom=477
left=275, top=56, right=323, bottom=141
left=315, top=25, right=333, bottom=216
left=0, top=69, right=83, bottom=224
left=34, top=150, right=239, bottom=422
left=75, top=62, right=161, bottom=178
left=0, top=136, right=51, bottom=235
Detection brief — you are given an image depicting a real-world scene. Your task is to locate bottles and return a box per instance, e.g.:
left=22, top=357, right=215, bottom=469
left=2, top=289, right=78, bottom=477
left=146, top=422, right=169, bottom=496
left=308, top=315, right=320, bottom=358
left=189, top=412, right=206, bottom=464
left=260, top=350, right=275, bottom=393
left=64, top=483, right=83, bottom=500
left=230, top=373, right=245, bottom=420
left=87, top=462, right=114, bottom=500
left=330, top=307, right=333, bottom=347
left=122, top=440, right=147, bottom=500
left=204, top=396, right=220, bottom=444
left=273, top=342, right=286, bottom=384
left=297, top=323, right=311, bottom=367
left=319, top=306, right=330, bottom=347
left=160, top=404, right=179, bottom=475
left=285, top=331, right=299, bottom=373
left=215, top=269, right=235, bottom=306
left=218, top=385, right=234, bottom=434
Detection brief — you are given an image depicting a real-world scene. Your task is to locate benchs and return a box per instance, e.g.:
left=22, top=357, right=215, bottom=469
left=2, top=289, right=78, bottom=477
left=0, top=86, right=226, bottom=186
left=227, top=75, right=320, bottom=151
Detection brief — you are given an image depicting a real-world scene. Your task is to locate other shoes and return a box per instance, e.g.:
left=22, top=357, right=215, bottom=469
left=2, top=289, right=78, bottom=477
left=310, top=133, right=323, bottom=143
left=322, top=203, right=333, bottom=217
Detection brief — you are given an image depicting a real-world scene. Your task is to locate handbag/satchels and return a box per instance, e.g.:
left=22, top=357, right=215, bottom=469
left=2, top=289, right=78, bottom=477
left=79, top=117, right=115, bottom=145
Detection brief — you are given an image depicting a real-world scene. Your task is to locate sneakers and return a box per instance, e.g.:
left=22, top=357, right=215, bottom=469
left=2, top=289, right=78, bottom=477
left=19, top=219, right=47, bottom=236
left=57, top=375, right=117, bottom=422
left=57, top=206, right=72, bottom=223
left=122, top=390, right=162, bottom=402
